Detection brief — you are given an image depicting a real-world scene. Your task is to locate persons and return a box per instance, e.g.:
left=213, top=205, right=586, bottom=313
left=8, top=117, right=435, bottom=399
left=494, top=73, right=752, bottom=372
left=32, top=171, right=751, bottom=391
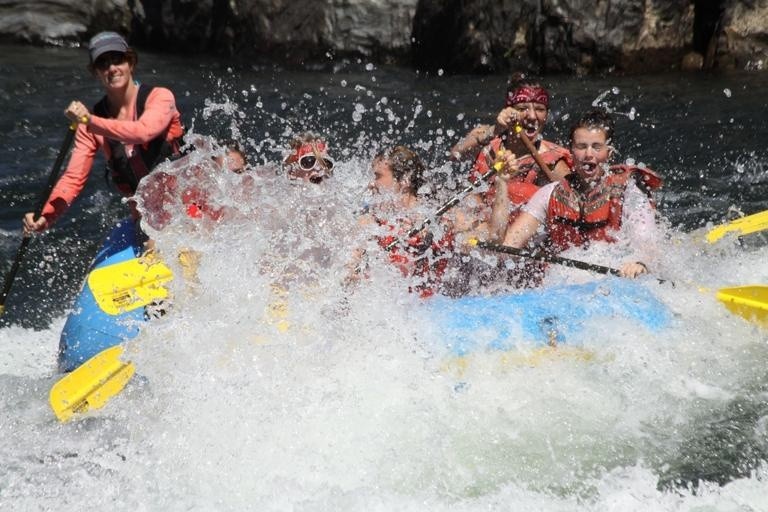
left=497, top=109, right=656, bottom=280
left=213, top=151, right=245, bottom=178
left=330, top=145, right=454, bottom=299
left=450, top=78, right=575, bottom=246
left=23, top=31, right=195, bottom=243
left=285, top=141, right=334, bottom=186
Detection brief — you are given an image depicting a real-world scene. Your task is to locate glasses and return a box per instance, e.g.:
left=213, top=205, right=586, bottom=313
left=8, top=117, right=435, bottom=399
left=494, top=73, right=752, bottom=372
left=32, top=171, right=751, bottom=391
left=298, top=152, right=336, bottom=173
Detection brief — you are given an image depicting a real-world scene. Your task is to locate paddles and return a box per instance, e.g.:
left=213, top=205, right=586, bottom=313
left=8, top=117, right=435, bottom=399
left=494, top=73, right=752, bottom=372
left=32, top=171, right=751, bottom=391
left=677, top=210, right=767, bottom=245
left=86, top=252, right=189, bottom=317
left=267, top=152, right=507, bottom=334
left=48, top=342, right=137, bottom=422
left=468, top=239, right=768, bottom=330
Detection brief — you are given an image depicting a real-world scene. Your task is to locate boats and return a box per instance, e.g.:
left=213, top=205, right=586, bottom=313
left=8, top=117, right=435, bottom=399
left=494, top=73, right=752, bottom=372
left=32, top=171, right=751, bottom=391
left=58, top=215, right=672, bottom=376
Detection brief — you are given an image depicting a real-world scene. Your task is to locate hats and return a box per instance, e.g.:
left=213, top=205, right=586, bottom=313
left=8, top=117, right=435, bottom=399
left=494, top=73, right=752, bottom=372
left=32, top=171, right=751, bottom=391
left=88, top=30, right=129, bottom=61
